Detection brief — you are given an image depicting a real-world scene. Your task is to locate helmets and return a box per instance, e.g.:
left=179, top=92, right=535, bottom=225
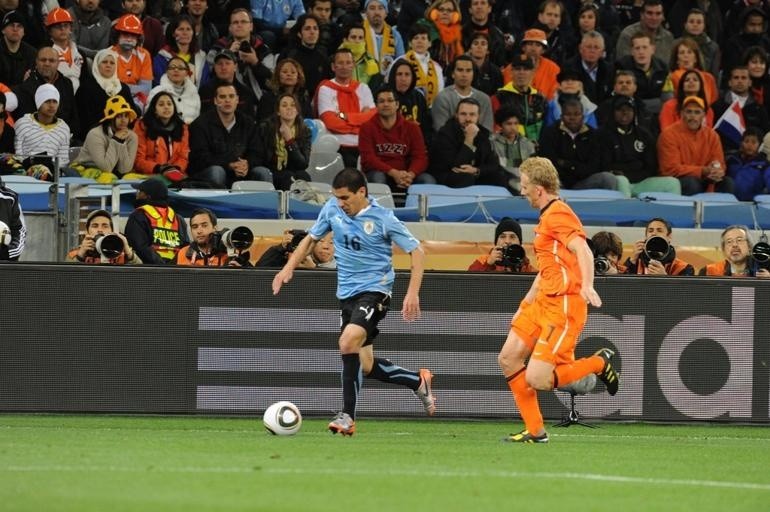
left=44, top=7, right=73, bottom=27
left=113, top=13, right=144, bottom=35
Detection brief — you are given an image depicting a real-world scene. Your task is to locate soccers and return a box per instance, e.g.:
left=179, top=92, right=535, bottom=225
left=263, top=401, right=302, bottom=435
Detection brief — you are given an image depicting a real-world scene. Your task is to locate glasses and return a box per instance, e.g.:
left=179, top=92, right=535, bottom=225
left=168, top=64, right=189, bottom=70
left=726, top=238, right=748, bottom=246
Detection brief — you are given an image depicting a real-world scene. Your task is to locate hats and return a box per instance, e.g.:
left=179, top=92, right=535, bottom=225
left=34, top=83, right=60, bottom=111
left=555, top=58, right=587, bottom=83
left=98, top=95, right=137, bottom=124
left=1, top=10, right=25, bottom=29
left=511, top=53, right=534, bottom=69
left=364, top=0, right=389, bottom=15
left=518, top=29, right=549, bottom=50
left=130, top=177, right=168, bottom=198
left=614, top=95, right=636, bottom=109
left=86, top=209, right=111, bottom=226
left=682, top=95, right=705, bottom=111
left=214, top=48, right=236, bottom=63
left=495, top=217, right=522, bottom=246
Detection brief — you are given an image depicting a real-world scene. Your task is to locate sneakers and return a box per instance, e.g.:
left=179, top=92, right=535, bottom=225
left=507, top=428, right=549, bottom=444
left=414, top=368, right=437, bottom=417
left=328, top=412, right=356, bottom=436
left=594, top=348, right=620, bottom=396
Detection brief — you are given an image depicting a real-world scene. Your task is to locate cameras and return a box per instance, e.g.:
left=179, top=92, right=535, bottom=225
left=494, top=243, right=526, bottom=268
left=234, top=41, right=252, bottom=58
left=594, top=257, right=610, bottom=275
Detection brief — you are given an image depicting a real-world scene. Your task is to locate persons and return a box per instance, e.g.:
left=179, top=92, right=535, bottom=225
left=0, top=1, right=770, bottom=279
left=497, top=157, right=620, bottom=443
left=272, top=169, right=436, bottom=434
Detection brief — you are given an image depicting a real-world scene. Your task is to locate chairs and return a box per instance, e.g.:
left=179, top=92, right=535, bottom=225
left=308, top=150, right=346, bottom=187
left=67, top=146, right=82, bottom=161
